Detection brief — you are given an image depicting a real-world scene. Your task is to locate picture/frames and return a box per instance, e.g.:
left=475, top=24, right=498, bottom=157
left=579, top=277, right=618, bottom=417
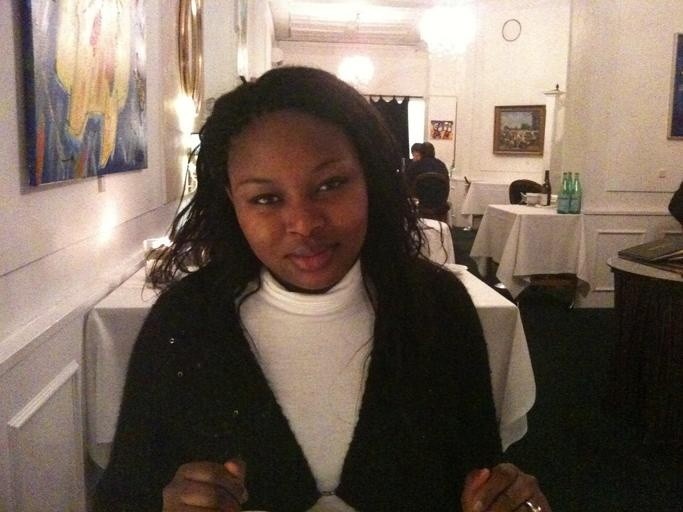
left=179, top=0, right=205, bottom=117
left=502, top=19, right=521, bottom=42
left=666, top=33, right=683, bottom=140
left=491, top=105, right=546, bottom=157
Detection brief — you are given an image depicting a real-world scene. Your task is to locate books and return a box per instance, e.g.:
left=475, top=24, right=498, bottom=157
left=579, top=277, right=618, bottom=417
left=617, top=234, right=683, bottom=274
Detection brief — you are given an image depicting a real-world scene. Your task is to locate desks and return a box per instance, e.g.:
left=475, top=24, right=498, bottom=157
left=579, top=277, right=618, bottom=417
left=606, top=254, right=683, bottom=477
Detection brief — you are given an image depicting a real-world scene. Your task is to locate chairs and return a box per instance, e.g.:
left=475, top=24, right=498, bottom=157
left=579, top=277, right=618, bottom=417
left=509, top=179, right=546, bottom=205
left=409, top=172, right=450, bottom=221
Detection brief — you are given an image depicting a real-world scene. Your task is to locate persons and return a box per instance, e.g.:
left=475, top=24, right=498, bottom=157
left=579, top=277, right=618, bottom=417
left=404, top=142, right=449, bottom=177
left=98, top=66, right=551, bottom=512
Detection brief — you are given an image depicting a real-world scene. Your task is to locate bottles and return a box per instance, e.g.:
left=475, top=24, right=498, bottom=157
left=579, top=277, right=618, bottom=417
left=557, top=172, right=580, bottom=214
left=540, top=170, right=551, bottom=206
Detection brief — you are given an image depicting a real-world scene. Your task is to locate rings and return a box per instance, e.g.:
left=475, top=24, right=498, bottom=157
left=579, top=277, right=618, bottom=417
left=525, top=500, right=542, bottom=512
